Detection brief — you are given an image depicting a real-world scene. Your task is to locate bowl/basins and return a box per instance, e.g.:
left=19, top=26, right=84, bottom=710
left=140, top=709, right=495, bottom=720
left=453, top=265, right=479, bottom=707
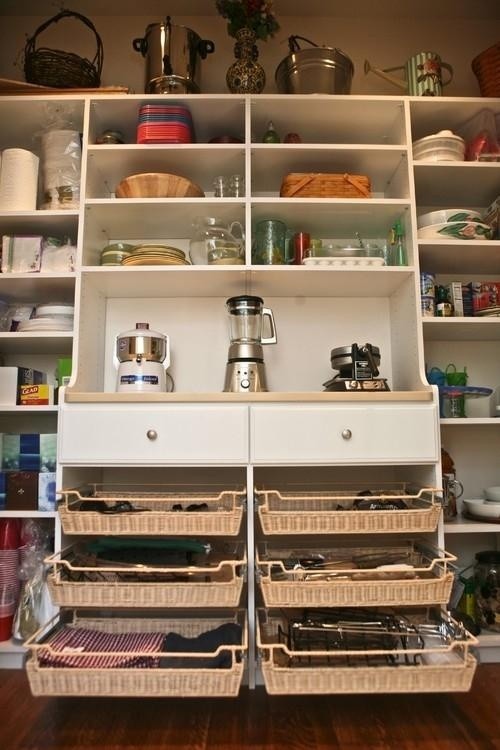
left=206, top=135, right=244, bottom=144
left=113, top=171, right=206, bottom=198
left=417, top=209, right=494, bottom=239
left=484, top=485, right=499, bottom=500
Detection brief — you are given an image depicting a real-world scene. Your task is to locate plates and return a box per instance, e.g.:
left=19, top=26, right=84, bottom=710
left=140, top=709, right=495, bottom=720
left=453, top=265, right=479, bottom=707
left=462, top=498, right=499, bottom=517
left=118, top=243, right=190, bottom=264
left=136, top=103, right=198, bottom=145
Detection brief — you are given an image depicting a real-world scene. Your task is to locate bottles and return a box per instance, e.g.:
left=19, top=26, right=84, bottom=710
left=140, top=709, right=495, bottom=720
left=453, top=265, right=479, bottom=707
left=263, top=119, right=279, bottom=143
left=212, top=173, right=244, bottom=197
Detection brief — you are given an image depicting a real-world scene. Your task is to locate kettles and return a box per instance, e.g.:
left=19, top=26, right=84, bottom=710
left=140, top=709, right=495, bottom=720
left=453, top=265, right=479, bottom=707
left=440, top=444, right=464, bottom=521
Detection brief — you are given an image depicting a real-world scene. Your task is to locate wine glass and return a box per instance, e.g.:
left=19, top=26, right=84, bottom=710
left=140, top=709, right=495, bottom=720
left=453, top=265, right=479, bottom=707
left=439, top=385, right=493, bottom=418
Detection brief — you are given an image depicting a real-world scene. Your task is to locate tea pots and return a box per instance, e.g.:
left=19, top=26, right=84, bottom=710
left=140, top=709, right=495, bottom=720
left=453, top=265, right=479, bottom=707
left=188, top=214, right=246, bottom=266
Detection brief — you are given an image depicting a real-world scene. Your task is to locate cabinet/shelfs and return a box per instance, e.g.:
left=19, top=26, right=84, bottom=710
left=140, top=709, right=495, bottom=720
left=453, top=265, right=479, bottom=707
left=402, top=89, right=499, bottom=665
left=50, top=90, right=455, bottom=712
left=0, top=89, right=111, bottom=671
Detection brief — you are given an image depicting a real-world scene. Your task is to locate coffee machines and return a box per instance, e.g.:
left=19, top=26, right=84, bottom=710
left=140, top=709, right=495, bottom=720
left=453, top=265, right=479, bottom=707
left=222, top=295, right=278, bottom=392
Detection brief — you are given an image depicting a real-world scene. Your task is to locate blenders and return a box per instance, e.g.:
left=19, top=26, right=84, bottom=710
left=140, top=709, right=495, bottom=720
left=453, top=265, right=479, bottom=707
left=112, top=322, right=172, bottom=392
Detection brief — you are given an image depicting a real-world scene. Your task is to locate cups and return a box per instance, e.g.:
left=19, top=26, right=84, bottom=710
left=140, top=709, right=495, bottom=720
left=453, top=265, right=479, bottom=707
left=311, top=237, right=323, bottom=256
left=428, top=371, right=467, bottom=419
left=294, top=231, right=310, bottom=264
left=255, top=219, right=296, bottom=265
left=0, top=516, right=43, bottom=638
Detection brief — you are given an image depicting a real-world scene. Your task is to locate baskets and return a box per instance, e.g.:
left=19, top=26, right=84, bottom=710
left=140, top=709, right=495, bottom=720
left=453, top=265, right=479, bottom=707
left=21, top=606, right=249, bottom=698
left=470, top=38, right=500, bottom=98
left=256, top=604, right=480, bottom=694
left=253, top=540, right=460, bottom=609
left=279, top=172, right=371, bottom=197
left=23, top=10, right=104, bottom=89
left=252, top=480, right=443, bottom=537
left=42, top=538, right=249, bottom=608
left=54, top=481, right=248, bottom=538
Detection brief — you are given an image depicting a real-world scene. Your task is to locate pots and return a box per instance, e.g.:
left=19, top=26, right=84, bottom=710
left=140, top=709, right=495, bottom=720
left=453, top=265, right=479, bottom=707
left=130, top=22, right=216, bottom=93
left=472, top=550, right=500, bottom=633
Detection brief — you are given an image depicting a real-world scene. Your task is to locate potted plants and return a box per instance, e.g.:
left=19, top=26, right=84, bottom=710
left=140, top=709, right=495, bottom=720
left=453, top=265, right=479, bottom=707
left=219, top=1, right=285, bottom=95
